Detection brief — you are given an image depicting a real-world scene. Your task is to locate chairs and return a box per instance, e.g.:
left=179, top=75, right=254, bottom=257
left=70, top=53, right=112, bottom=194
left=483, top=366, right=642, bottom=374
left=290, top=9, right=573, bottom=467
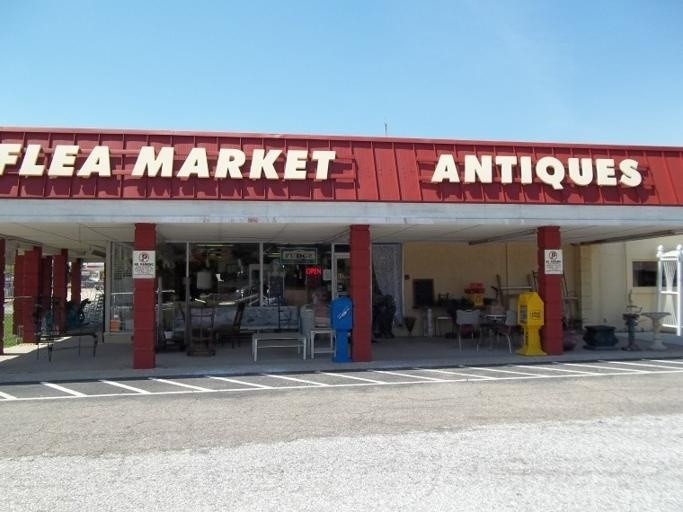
left=213, top=300, right=245, bottom=348
left=455, top=308, right=523, bottom=353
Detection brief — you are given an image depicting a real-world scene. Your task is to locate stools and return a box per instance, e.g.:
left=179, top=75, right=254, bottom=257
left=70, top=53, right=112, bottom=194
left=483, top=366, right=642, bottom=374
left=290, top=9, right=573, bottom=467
left=307, top=327, right=336, bottom=360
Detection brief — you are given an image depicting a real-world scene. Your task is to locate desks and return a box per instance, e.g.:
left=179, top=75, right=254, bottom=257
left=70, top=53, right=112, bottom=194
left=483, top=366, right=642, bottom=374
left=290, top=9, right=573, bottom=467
left=32, top=332, right=97, bottom=362
left=252, top=329, right=306, bottom=360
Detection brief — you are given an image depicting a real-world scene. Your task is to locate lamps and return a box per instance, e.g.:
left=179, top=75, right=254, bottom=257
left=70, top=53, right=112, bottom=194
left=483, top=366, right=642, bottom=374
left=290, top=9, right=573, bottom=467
left=196, top=272, right=211, bottom=297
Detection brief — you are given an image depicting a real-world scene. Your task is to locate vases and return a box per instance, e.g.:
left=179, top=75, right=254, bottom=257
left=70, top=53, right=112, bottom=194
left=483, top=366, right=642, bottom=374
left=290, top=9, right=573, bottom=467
left=373, top=293, right=396, bottom=333
